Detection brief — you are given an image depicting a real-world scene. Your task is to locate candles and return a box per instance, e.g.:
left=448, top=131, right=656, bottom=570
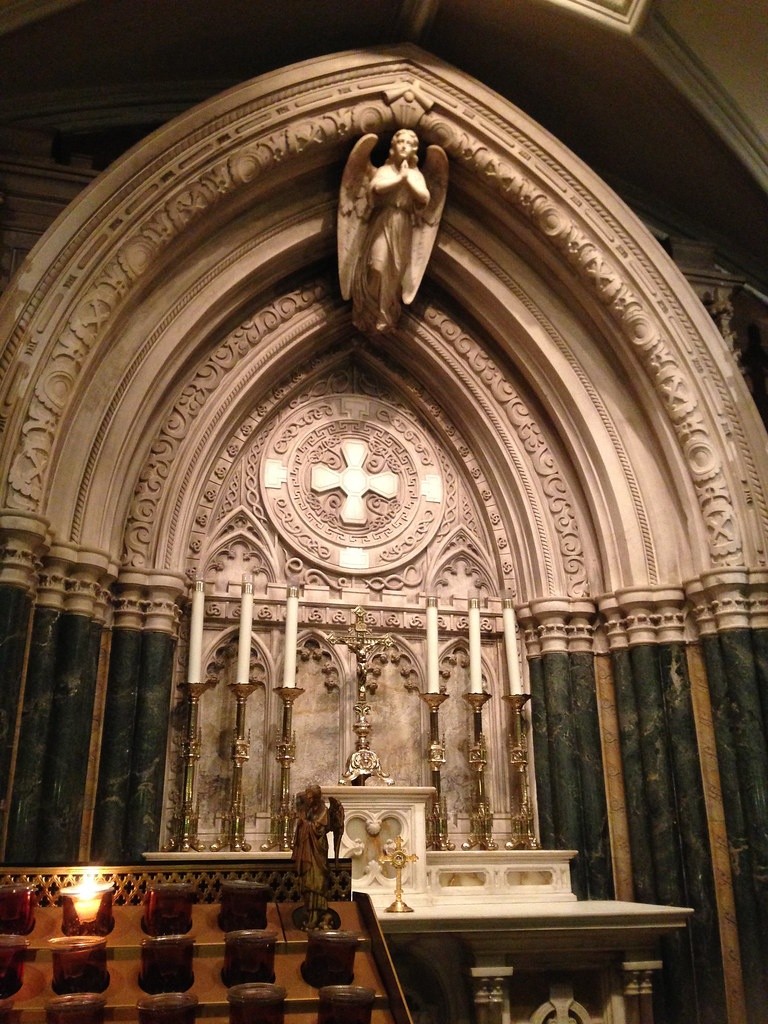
left=227, top=983, right=288, bottom=1024
left=282, top=584, right=300, bottom=688
left=225, top=929, right=277, bottom=988
left=61, top=885, right=116, bottom=937
left=136, top=993, right=198, bottom=1024
left=426, top=596, right=439, bottom=693
left=502, top=597, right=522, bottom=696
left=48, top=936, right=108, bottom=994
left=188, top=579, right=204, bottom=683
left=45, top=992, right=106, bottom=1024
left=220, top=880, right=269, bottom=931
left=307, top=929, right=357, bottom=984
left=0, top=934, right=31, bottom=992
left=318, top=985, right=377, bottom=1024
left=0, top=884, right=36, bottom=936
left=140, top=934, right=194, bottom=994
left=471, top=598, right=481, bottom=694
left=145, top=882, right=192, bottom=934
left=237, top=583, right=255, bottom=684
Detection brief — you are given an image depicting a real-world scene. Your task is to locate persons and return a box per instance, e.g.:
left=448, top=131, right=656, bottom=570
left=294, top=785, right=345, bottom=927
left=337, top=129, right=448, bottom=335
